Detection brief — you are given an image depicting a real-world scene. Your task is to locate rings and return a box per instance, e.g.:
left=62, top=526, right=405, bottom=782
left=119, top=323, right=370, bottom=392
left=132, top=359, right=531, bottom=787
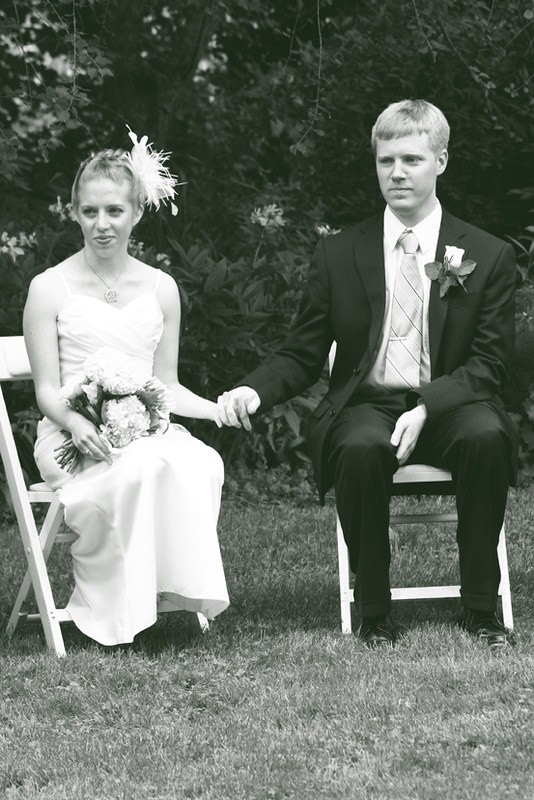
left=84, top=450, right=90, bottom=456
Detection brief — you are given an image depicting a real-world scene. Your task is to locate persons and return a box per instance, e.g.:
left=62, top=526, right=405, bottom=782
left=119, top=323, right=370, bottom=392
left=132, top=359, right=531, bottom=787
left=214, top=100, right=517, bottom=649
left=23, top=132, right=239, bottom=646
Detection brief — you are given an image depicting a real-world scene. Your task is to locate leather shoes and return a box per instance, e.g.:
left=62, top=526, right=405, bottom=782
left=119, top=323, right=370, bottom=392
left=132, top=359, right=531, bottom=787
left=363, top=616, right=395, bottom=648
left=464, top=610, right=516, bottom=645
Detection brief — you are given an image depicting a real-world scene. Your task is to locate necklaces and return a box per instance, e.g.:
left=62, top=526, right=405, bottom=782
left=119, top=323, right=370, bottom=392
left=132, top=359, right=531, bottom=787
left=83, top=252, right=126, bottom=303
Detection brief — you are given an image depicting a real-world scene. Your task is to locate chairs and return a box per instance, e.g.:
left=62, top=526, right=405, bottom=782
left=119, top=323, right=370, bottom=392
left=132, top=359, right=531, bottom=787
left=0, top=337, right=209, bottom=657
left=327, top=340, right=515, bottom=635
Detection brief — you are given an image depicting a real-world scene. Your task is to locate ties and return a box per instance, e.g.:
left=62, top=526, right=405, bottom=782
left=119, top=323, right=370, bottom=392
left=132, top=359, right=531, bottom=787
left=383, top=231, right=424, bottom=393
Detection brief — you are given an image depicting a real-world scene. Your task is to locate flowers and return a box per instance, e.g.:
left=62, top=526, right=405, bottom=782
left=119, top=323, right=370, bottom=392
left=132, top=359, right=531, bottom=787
left=425, top=246, right=478, bottom=299
left=53, top=369, right=170, bottom=475
